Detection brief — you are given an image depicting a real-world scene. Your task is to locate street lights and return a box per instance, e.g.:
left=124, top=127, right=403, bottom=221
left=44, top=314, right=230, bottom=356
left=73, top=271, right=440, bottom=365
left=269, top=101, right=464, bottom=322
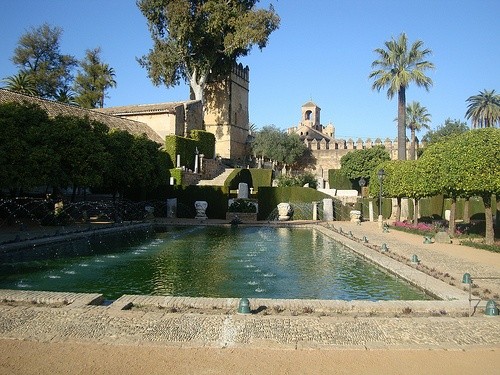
left=376, top=168, right=386, bottom=214
